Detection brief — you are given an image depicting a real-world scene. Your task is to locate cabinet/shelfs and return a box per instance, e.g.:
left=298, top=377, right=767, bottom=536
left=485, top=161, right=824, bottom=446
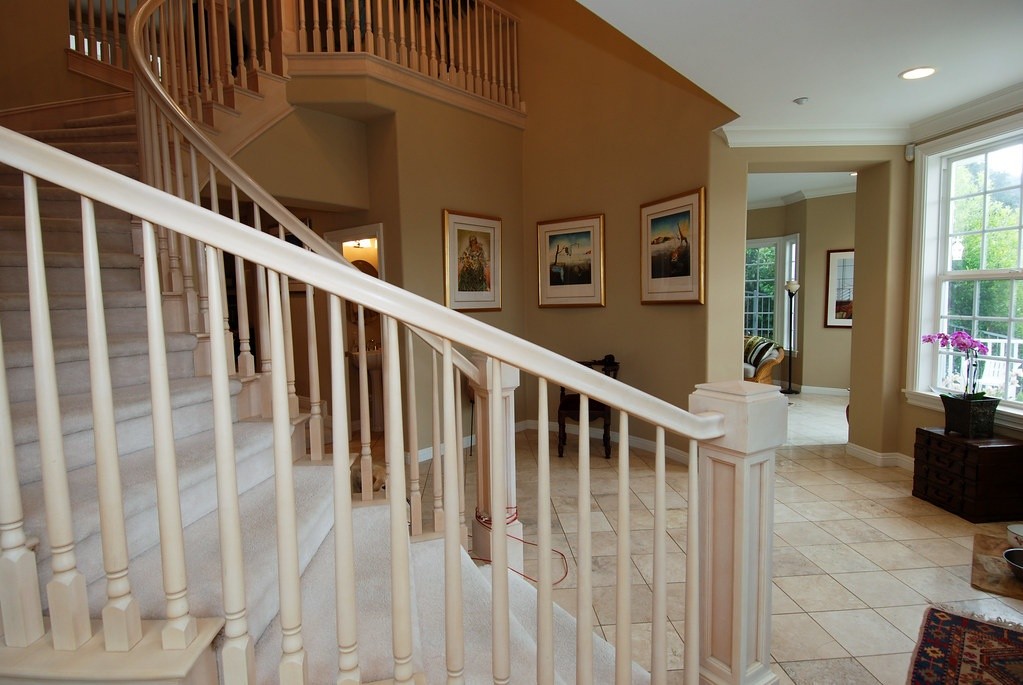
left=912, top=426, right=1023, bottom=523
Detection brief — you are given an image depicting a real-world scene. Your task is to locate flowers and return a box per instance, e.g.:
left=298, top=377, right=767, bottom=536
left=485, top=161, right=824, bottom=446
left=922, top=330, right=989, bottom=399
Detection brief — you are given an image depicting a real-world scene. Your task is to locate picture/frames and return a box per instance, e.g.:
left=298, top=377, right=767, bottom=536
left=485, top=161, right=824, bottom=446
left=267, top=216, right=312, bottom=294
left=640, top=185, right=706, bottom=304
left=823, top=248, right=854, bottom=328
left=441, top=207, right=502, bottom=313
left=536, top=214, right=606, bottom=308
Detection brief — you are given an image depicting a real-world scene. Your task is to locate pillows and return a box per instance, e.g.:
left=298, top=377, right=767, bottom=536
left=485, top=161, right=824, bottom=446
left=747, top=341, right=775, bottom=366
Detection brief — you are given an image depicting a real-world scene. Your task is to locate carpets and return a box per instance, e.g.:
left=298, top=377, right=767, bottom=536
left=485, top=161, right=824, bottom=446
left=970, top=532, right=1023, bottom=601
left=906, top=601, right=1023, bottom=685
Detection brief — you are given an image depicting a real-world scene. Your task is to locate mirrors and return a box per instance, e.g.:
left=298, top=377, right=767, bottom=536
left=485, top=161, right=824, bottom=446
left=345, top=260, right=379, bottom=326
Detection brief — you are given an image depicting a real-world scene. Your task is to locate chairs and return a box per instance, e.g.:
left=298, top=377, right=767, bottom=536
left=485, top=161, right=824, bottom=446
left=743, top=336, right=785, bottom=384
left=558, top=353, right=620, bottom=458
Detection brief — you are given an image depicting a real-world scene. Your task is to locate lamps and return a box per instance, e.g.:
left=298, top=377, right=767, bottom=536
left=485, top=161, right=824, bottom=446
left=781, top=280, right=801, bottom=394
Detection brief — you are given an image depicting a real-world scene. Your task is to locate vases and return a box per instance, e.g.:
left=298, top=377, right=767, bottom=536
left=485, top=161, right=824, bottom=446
left=941, top=393, right=1001, bottom=437
